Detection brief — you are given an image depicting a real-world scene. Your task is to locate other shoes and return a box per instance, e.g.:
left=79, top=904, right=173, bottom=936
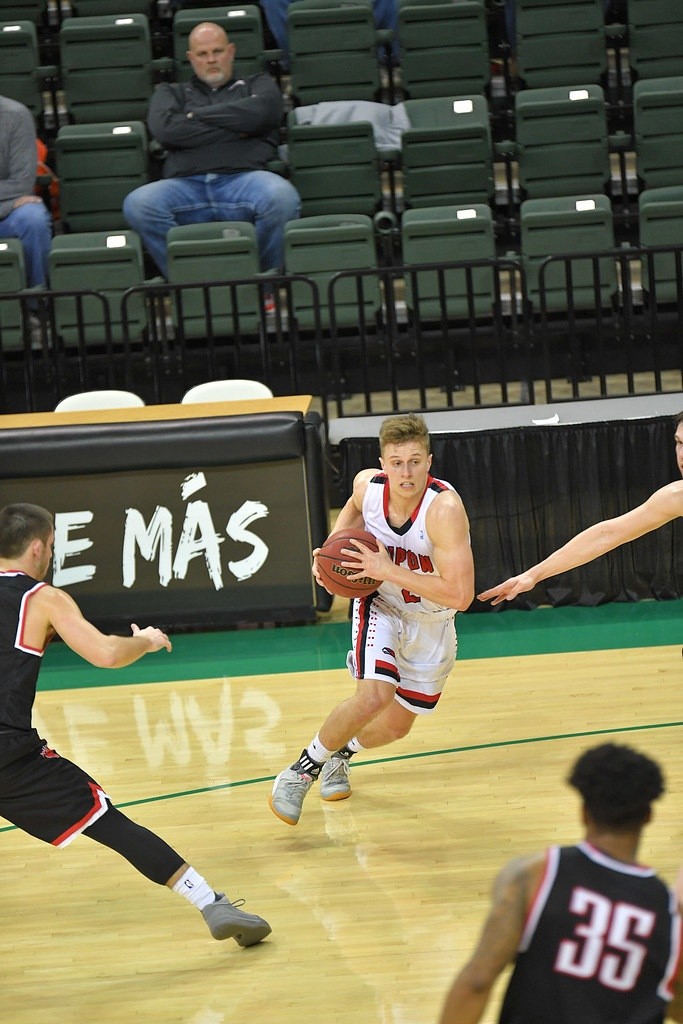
left=29, top=310, right=53, bottom=350
left=146, top=295, right=176, bottom=341
left=261, top=294, right=288, bottom=333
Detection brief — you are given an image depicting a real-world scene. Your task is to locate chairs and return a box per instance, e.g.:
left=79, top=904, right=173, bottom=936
left=0, top=21, right=59, bottom=143
left=0, top=238, right=45, bottom=348
left=285, top=0, right=395, bottom=104
left=55, top=121, right=149, bottom=233
left=167, top=221, right=261, bottom=335
left=284, top=214, right=382, bottom=327
left=618, top=0, right=683, bottom=84
left=498, top=0, right=617, bottom=95
left=521, top=194, right=619, bottom=309
left=514, top=85, right=631, bottom=201
left=288, top=100, right=396, bottom=219
left=174, top=4, right=285, bottom=97
left=637, top=187, right=683, bottom=303
left=391, top=0, right=492, bottom=99
left=60, top=13, right=173, bottom=126
left=402, top=204, right=497, bottom=318
left=633, top=77, right=683, bottom=190
left=400, top=95, right=514, bottom=210
left=47, top=230, right=165, bottom=347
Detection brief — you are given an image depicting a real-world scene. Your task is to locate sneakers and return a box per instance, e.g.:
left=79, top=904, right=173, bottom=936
left=320, top=757, right=352, bottom=801
left=269, top=766, right=314, bottom=825
left=199, top=893, right=272, bottom=947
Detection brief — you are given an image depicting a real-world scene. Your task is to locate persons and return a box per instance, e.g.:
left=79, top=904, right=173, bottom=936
left=0, top=96, right=54, bottom=352
left=270, top=416, right=473, bottom=827
left=440, top=743, right=683, bottom=1024
left=1, top=500, right=272, bottom=947
left=123, top=23, right=300, bottom=316
left=260, top=0, right=399, bottom=61
left=480, top=413, right=683, bottom=606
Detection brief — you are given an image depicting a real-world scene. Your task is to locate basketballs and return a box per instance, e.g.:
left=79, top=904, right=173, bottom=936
left=316, top=528, right=384, bottom=599
